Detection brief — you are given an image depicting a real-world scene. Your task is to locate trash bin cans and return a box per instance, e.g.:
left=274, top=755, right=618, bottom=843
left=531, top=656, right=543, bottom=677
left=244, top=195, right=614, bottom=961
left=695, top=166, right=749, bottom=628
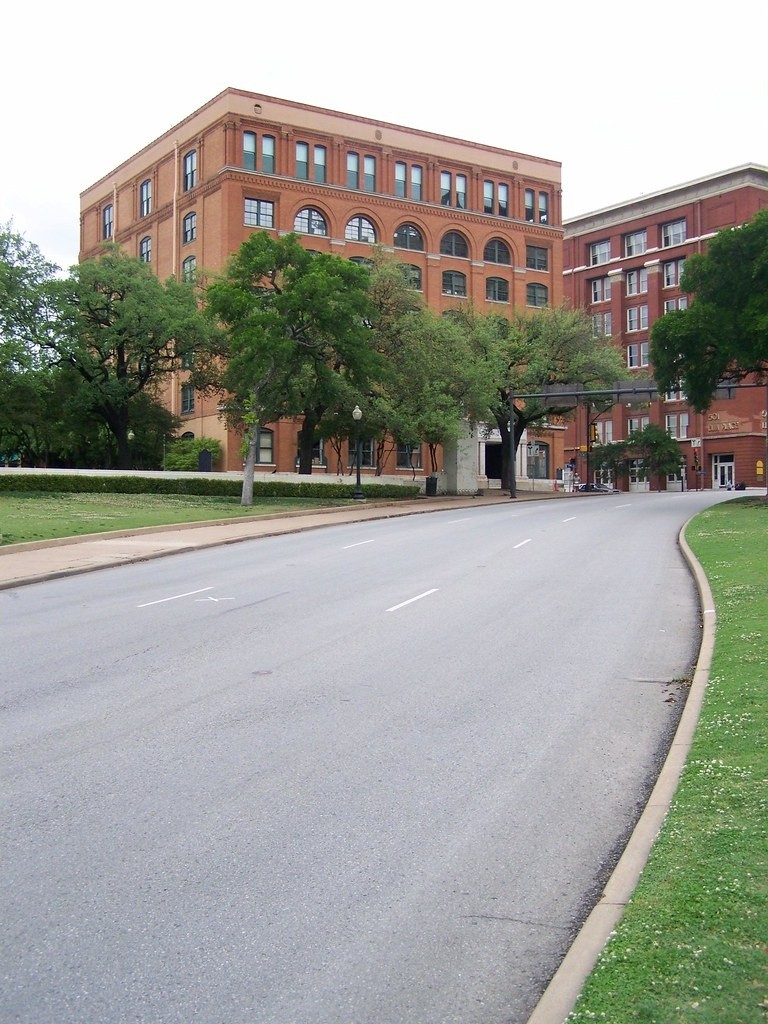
left=198, top=449, right=212, bottom=472
left=426, top=477, right=438, bottom=496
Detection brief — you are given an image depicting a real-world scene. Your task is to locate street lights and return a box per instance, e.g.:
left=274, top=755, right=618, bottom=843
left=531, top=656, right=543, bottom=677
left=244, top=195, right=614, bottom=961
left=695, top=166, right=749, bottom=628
left=352, top=405, right=365, bottom=499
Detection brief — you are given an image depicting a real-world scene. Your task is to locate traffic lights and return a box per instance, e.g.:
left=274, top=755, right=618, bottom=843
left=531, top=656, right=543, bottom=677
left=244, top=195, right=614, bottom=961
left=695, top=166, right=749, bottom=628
left=693, top=448, right=699, bottom=463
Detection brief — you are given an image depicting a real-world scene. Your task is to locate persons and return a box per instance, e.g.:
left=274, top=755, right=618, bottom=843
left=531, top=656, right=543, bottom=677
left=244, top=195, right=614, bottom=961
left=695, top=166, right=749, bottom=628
left=573, top=473, right=581, bottom=492
left=727, top=478, right=744, bottom=491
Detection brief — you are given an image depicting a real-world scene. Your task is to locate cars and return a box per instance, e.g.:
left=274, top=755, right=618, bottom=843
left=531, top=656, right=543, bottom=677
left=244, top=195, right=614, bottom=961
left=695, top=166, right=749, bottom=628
left=578, top=482, right=620, bottom=493
